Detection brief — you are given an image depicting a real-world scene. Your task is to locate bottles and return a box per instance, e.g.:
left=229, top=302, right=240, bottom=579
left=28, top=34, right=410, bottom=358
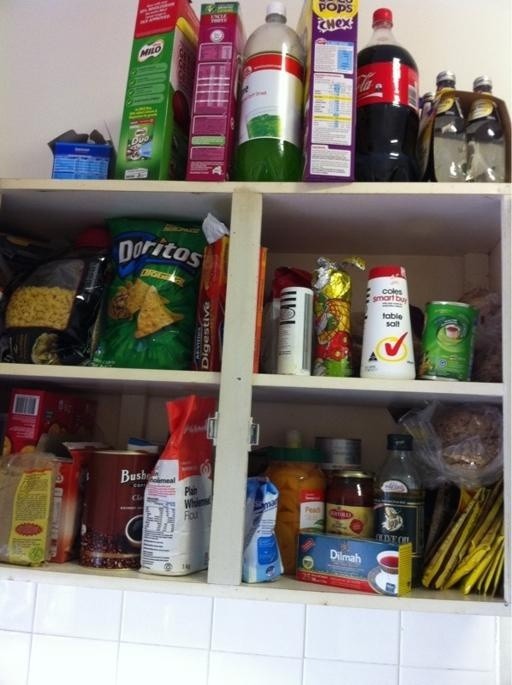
left=358, top=264, right=417, bottom=379
left=466, top=74, right=506, bottom=183
left=418, top=91, right=433, bottom=123
left=355, top=7, right=420, bottom=183
left=230, top=0, right=307, bottom=181
left=431, top=71, right=468, bottom=182
left=374, top=432, right=426, bottom=589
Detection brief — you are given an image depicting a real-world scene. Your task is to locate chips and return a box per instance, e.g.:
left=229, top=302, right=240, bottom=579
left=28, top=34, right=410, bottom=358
left=124, top=277, right=185, bottom=340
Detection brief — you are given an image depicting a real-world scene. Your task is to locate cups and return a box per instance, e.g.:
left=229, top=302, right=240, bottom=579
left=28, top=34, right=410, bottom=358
left=375, top=550, right=401, bottom=575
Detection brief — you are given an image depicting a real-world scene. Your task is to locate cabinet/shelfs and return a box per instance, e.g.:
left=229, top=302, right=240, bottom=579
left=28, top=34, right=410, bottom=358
left=0, top=177, right=512, bottom=617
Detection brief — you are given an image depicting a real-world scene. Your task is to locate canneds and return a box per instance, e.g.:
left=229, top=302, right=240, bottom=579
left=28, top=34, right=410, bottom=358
left=78, top=449, right=156, bottom=571
left=325, top=468, right=374, bottom=539
left=419, top=300, right=476, bottom=380
left=256, top=448, right=325, bottom=574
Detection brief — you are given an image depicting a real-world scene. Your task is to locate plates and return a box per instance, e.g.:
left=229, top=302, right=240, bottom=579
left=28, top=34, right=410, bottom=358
left=367, top=566, right=399, bottom=597
